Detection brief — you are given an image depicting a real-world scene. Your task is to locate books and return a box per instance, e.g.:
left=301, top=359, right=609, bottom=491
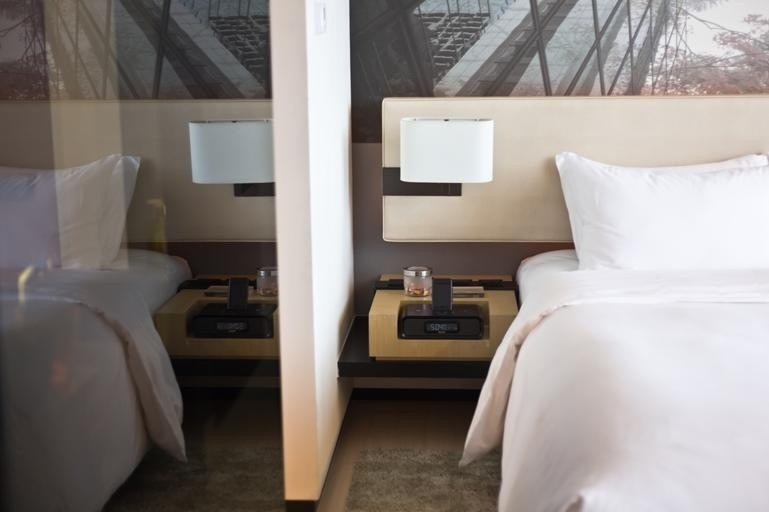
left=204, top=285, right=254, bottom=297
left=451, top=286, right=487, bottom=302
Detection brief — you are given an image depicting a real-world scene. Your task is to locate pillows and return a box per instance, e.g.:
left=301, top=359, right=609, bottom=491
left=1, top=153, right=143, bottom=272
left=553, top=151, right=769, bottom=269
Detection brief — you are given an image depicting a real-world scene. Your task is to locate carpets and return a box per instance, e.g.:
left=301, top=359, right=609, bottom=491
left=116, top=443, right=290, bottom=512
left=340, top=446, right=502, bottom=512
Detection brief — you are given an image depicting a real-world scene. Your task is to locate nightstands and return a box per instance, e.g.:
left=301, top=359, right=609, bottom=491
left=154, top=272, right=279, bottom=392
left=337, top=271, right=521, bottom=402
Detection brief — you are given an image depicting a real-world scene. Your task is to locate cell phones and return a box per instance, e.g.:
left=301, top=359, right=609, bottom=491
left=226, top=276, right=249, bottom=316
left=432, top=276, right=454, bottom=312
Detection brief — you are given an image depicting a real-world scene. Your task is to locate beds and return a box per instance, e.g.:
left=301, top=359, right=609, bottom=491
left=0, top=250, right=194, bottom=511
left=516, top=251, right=769, bottom=510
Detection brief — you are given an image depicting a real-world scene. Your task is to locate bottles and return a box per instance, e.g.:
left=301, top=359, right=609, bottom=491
left=256, top=265, right=278, bottom=297
left=403, top=265, right=433, bottom=297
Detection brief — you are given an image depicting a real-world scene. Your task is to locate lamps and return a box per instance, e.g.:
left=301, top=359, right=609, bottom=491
left=398, top=115, right=497, bottom=187
left=188, top=119, right=273, bottom=184
